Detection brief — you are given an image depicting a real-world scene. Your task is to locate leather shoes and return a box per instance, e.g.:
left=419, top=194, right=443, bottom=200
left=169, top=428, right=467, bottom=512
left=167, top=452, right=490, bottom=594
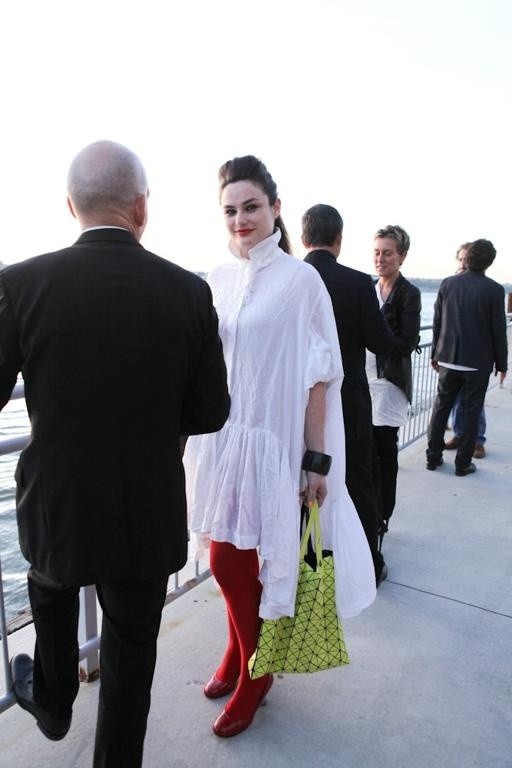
left=473, top=441, right=488, bottom=462
left=454, top=461, right=476, bottom=478
left=442, top=435, right=463, bottom=452
left=376, top=564, right=388, bottom=588
left=427, top=456, right=445, bottom=472
left=6, top=651, right=72, bottom=743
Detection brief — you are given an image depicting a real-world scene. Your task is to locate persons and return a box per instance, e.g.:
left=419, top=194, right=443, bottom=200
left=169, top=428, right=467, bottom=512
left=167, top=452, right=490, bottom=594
left=184, top=156, right=378, bottom=737
left=445, top=241, right=486, bottom=458
left=0, top=140, right=231, bottom=768
left=298, top=205, right=398, bottom=590
left=426, top=239, right=509, bottom=476
left=365, top=225, right=421, bottom=535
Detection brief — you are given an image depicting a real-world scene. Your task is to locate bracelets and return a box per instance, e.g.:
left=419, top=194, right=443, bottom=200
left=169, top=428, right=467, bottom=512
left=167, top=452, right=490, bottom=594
left=300, top=450, right=333, bottom=478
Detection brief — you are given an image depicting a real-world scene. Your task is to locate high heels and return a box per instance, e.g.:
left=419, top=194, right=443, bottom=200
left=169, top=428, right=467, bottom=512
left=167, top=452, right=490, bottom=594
left=203, top=671, right=240, bottom=699
left=212, top=669, right=275, bottom=739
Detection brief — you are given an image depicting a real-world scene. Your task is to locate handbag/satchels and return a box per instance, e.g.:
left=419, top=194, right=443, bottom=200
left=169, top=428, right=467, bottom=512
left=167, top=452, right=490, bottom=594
left=247, top=495, right=352, bottom=681
